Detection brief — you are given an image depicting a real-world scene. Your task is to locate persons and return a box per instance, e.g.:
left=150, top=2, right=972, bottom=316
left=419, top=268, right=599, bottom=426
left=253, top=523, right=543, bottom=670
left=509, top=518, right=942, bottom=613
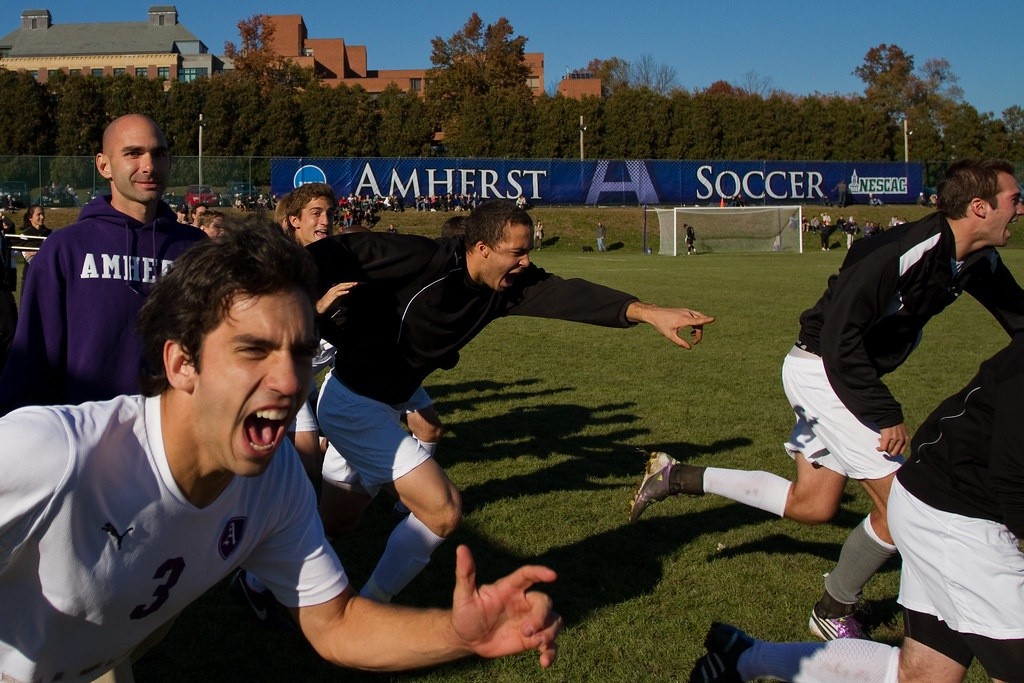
left=0, top=102, right=717, bottom=683
left=689, top=333, right=1023, bottom=683
left=790, top=178, right=938, bottom=251
left=625, top=157, right=1024, bottom=645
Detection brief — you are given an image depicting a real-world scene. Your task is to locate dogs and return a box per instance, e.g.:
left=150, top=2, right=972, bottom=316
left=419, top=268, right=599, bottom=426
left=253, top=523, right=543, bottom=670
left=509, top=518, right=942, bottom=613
left=583, top=246, right=594, bottom=252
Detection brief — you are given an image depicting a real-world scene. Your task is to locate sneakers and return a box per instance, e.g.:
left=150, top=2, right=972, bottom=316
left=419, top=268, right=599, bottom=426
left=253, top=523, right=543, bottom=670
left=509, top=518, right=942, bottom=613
left=810, top=606, right=871, bottom=643
left=631, top=451, right=683, bottom=520
left=691, top=621, right=753, bottom=682
